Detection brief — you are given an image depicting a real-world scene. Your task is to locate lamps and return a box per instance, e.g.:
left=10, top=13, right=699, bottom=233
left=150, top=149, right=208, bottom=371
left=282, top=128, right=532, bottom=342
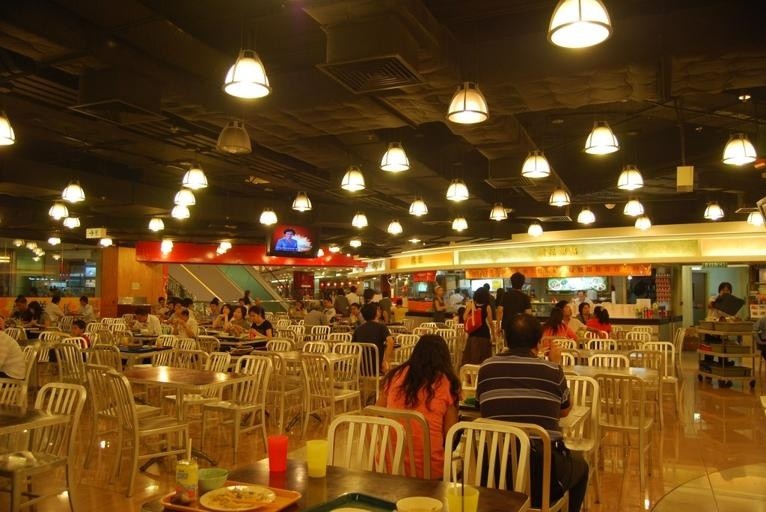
left=0, top=90, right=766, bottom=263
left=547, top=0, right=612, bottom=49
left=446, top=20, right=490, bottom=127
left=221, top=15, right=274, bottom=101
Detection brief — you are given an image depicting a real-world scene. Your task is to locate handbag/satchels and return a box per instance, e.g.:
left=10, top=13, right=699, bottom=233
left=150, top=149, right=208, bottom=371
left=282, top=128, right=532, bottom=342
left=463, top=299, right=483, bottom=334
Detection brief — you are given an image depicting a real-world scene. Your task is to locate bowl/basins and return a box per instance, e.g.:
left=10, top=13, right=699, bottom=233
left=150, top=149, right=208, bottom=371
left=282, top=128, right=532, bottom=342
left=198, top=467, right=228, bottom=492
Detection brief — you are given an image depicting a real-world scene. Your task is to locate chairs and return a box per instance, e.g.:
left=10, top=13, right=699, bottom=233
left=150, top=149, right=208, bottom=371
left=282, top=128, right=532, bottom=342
left=0, top=306, right=688, bottom=512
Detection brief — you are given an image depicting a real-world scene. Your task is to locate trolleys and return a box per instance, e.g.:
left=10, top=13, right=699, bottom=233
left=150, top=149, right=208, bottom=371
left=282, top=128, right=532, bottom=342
left=693, top=381, right=761, bottom=461
left=691, top=320, right=757, bottom=389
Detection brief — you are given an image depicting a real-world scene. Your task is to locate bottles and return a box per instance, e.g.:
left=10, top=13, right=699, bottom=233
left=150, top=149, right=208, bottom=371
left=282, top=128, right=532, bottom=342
left=634, top=302, right=659, bottom=320
left=156, top=337, right=162, bottom=348
left=655, top=272, right=671, bottom=303
left=25, top=272, right=67, bottom=292
left=161, top=325, right=168, bottom=334
left=249, top=328, right=256, bottom=341
left=59, top=262, right=97, bottom=293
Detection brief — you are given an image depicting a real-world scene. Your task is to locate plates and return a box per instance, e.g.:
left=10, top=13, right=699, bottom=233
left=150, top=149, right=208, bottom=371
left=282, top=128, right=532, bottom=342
left=457, top=399, right=475, bottom=408
left=200, top=485, right=277, bottom=512
left=126, top=343, right=144, bottom=349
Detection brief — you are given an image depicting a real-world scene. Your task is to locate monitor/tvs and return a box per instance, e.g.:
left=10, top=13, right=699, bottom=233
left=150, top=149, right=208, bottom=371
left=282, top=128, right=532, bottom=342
left=265, top=221, right=320, bottom=257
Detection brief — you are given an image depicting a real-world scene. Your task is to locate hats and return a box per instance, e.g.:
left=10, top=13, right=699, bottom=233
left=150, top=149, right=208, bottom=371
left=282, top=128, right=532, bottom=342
left=434, top=286, right=440, bottom=291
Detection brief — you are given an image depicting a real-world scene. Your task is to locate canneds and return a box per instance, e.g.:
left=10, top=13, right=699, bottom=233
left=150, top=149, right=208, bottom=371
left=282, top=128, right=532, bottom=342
left=176, top=459, right=198, bottom=503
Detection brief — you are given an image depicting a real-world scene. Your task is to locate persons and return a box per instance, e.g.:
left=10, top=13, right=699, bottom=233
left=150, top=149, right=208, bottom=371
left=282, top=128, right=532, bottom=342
left=282, top=282, right=337, bottom=341
left=74, top=296, right=97, bottom=323
left=60, top=319, right=90, bottom=362
left=210, top=290, right=274, bottom=372
left=391, top=298, right=408, bottom=325
left=2, top=331, right=26, bottom=381
left=541, top=300, right=612, bottom=360
left=156, top=297, right=198, bottom=363
left=453, top=307, right=467, bottom=326
left=28, top=285, right=74, bottom=297
left=433, top=285, right=449, bottom=324
left=11, top=294, right=52, bottom=339
left=273, top=228, right=298, bottom=252
left=699, top=282, right=740, bottom=387
left=367, top=334, right=466, bottom=481
left=574, top=290, right=598, bottom=305
left=463, top=274, right=531, bottom=347
left=334, top=286, right=392, bottom=328
left=352, top=303, right=390, bottom=377
left=122, top=307, right=163, bottom=365
left=46, top=294, right=66, bottom=327
left=449, top=288, right=471, bottom=306
left=475, top=312, right=589, bottom=511
left=753, top=316, right=766, bottom=361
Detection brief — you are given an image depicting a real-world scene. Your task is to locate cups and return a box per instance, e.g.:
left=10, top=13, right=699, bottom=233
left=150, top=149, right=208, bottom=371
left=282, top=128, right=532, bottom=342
left=139, top=328, right=149, bottom=336
left=306, top=439, right=329, bottom=478
left=269, top=470, right=288, bottom=491
left=305, top=476, right=328, bottom=509
left=446, top=485, right=480, bottom=512
left=396, top=495, right=444, bottom=512
left=611, top=290, right=616, bottom=304
left=267, top=435, right=289, bottom=473
